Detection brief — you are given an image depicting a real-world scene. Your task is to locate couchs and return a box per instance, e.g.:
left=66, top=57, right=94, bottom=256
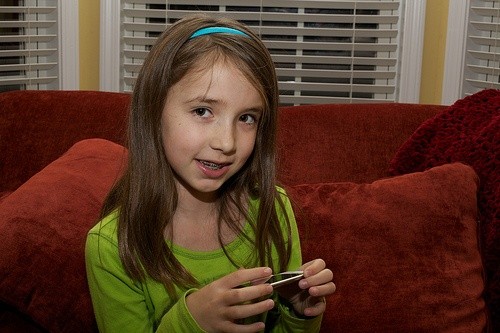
left=0, top=90, right=500, bottom=332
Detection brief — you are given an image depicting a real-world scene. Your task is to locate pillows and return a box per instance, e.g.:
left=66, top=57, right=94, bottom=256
left=283, top=161, right=489, bottom=333
left=0, top=138, right=128, bottom=333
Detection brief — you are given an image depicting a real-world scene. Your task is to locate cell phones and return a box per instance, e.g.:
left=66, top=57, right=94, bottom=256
left=233, top=271, right=305, bottom=289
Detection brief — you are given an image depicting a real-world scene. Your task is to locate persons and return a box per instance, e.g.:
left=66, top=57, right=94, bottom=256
left=84, top=14, right=337, bottom=333
